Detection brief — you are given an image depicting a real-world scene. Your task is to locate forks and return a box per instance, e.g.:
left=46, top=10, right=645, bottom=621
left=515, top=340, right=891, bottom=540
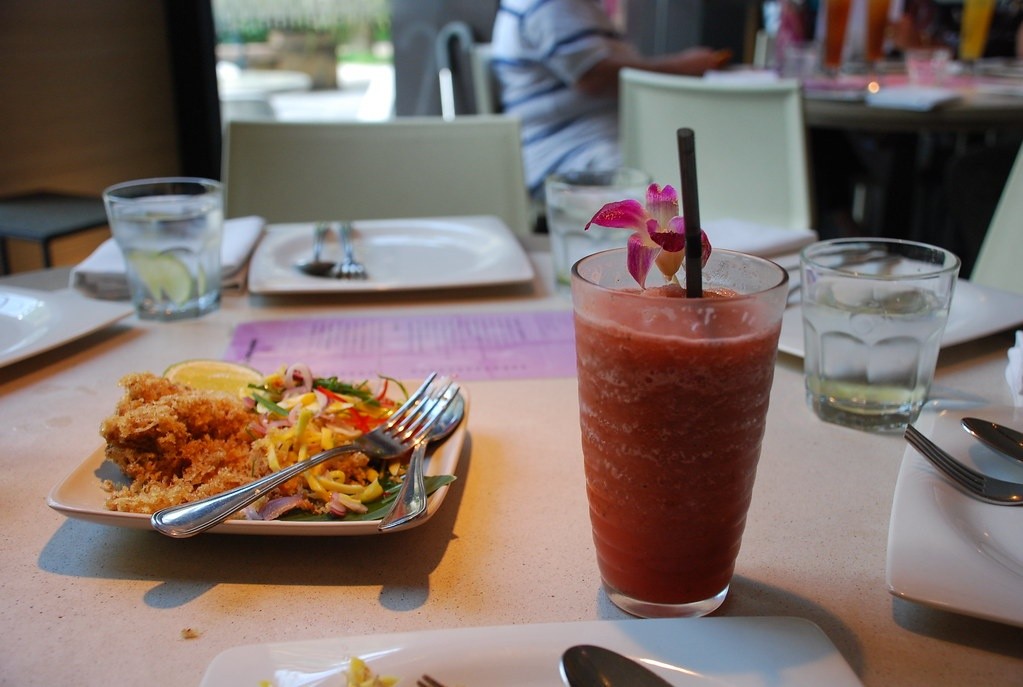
left=152, top=373, right=460, bottom=541
left=335, top=220, right=365, bottom=277
left=905, top=423, right=1023, bottom=506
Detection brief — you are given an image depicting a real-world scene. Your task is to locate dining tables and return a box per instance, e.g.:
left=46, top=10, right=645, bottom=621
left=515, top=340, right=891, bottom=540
left=0, top=238, right=1023, bottom=687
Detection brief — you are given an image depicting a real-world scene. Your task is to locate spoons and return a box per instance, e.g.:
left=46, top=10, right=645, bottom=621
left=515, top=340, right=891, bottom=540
left=296, top=218, right=334, bottom=271
left=559, top=644, right=673, bottom=687
left=378, top=387, right=467, bottom=527
left=962, top=417, right=1023, bottom=464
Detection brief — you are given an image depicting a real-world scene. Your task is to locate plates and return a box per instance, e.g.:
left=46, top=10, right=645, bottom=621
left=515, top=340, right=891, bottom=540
left=248, top=217, right=534, bottom=291
left=756, top=241, right=1023, bottom=364
left=199, top=613, right=865, bottom=687
left=49, top=380, right=467, bottom=533
left=0, top=284, right=132, bottom=366
left=886, top=400, right=1023, bottom=623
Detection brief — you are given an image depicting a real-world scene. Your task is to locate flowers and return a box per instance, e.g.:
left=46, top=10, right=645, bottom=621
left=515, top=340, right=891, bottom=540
left=582, top=182, right=712, bottom=292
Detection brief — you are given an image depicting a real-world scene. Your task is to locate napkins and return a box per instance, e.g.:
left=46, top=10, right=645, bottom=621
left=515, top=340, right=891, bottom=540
left=70, top=215, right=263, bottom=300
left=869, top=87, right=960, bottom=109
left=703, top=221, right=819, bottom=286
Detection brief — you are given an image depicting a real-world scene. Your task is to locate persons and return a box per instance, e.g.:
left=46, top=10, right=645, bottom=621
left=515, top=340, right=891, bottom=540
left=491, top=0, right=735, bottom=203
left=762, top=0, right=1023, bottom=279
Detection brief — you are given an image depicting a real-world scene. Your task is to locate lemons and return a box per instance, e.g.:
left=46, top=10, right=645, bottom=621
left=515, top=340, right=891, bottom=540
left=163, top=358, right=265, bottom=409
left=126, top=248, right=192, bottom=305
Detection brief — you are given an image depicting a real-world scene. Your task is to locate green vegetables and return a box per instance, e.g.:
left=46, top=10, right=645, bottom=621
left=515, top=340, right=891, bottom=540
left=247, top=375, right=457, bottom=521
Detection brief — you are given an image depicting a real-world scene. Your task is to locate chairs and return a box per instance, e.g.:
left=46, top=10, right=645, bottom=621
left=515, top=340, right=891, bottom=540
left=436, top=19, right=480, bottom=119
left=221, top=118, right=534, bottom=247
left=467, top=43, right=505, bottom=119
left=616, top=70, right=811, bottom=243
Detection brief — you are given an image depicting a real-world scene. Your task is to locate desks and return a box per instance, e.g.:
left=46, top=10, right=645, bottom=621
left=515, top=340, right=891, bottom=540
left=689, top=63, right=1022, bottom=263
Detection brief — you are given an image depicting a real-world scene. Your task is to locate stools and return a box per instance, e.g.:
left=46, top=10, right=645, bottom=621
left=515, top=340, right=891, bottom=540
left=0, top=188, right=106, bottom=278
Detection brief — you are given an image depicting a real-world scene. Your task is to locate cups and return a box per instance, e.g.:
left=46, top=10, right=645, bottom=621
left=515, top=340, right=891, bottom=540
left=782, top=43, right=822, bottom=78
left=908, top=50, right=951, bottom=86
left=572, top=245, right=789, bottom=619
left=804, top=239, right=958, bottom=432
left=544, top=170, right=654, bottom=287
left=103, top=176, right=225, bottom=320
